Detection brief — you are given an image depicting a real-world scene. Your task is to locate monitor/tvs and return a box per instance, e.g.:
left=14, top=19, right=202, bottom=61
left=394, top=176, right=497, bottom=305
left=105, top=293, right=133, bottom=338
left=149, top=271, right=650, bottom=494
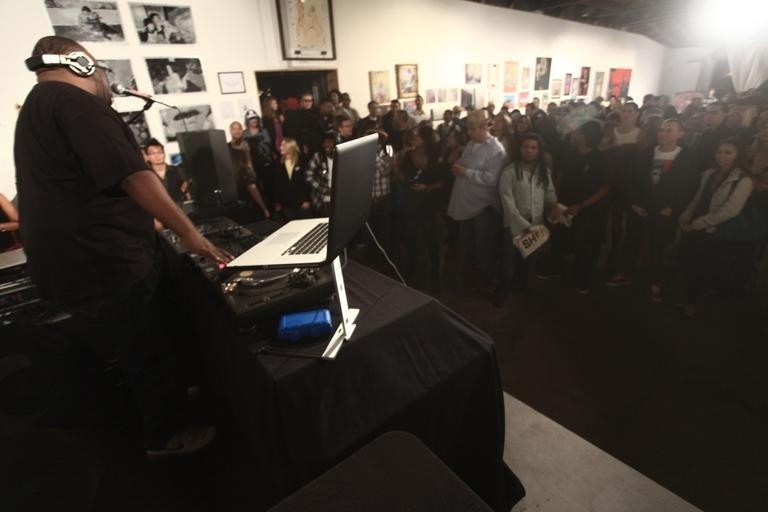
left=225, top=133, right=379, bottom=268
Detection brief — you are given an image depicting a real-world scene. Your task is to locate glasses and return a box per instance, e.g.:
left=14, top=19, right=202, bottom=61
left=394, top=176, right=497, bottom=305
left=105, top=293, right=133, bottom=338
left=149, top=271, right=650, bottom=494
left=148, top=151, right=164, bottom=155
left=341, top=125, right=353, bottom=128
left=301, top=99, right=313, bottom=102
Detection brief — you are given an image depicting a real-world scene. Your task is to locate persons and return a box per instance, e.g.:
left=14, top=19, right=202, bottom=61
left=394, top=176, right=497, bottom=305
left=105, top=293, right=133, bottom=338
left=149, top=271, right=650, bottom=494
left=14, top=36, right=235, bottom=394
left=227, top=121, right=263, bottom=178
left=266, top=137, right=313, bottom=221
left=510, top=115, right=532, bottom=149
left=306, top=133, right=336, bottom=216
left=490, top=113, right=514, bottom=142
left=452, top=106, right=464, bottom=126
left=261, top=96, right=284, bottom=159
left=144, top=138, right=192, bottom=231
left=319, top=99, right=335, bottom=132
left=383, top=99, right=401, bottom=132
left=613, top=102, right=641, bottom=145
left=288, top=91, right=331, bottom=141
left=487, top=95, right=587, bottom=127
left=351, top=100, right=382, bottom=137
left=340, top=93, right=360, bottom=124
left=243, top=109, right=274, bottom=167
left=408, top=95, right=430, bottom=126
left=391, top=125, right=453, bottom=282
left=0, top=193, right=22, bottom=232
left=570, top=142, right=654, bottom=286
left=693, top=102, right=735, bottom=168
left=446, top=109, right=508, bottom=302
left=230, top=140, right=270, bottom=222
left=680, top=137, right=754, bottom=299
left=336, top=117, right=354, bottom=142
left=691, top=88, right=768, bottom=118
left=567, top=119, right=608, bottom=291
left=595, top=94, right=680, bottom=121
left=410, top=120, right=433, bottom=169
left=743, top=109, right=768, bottom=190
left=608, top=118, right=701, bottom=300
left=419, top=128, right=446, bottom=171
left=438, top=109, right=461, bottom=141
left=328, top=89, right=355, bottom=125
left=387, top=109, right=414, bottom=153
left=499, top=130, right=577, bottom=290
left=369, top=132, right=392, bottom=217
left=461, top=104, right=476, bottom=123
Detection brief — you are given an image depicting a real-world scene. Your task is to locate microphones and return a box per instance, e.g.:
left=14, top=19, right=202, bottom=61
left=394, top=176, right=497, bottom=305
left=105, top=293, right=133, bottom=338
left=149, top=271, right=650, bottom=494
left=110, top=82, right=175, bottom=108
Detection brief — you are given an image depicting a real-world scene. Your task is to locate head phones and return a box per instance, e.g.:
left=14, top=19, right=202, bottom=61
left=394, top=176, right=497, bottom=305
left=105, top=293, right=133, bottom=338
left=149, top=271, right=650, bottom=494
left=26, top=52, right=95, bottom=78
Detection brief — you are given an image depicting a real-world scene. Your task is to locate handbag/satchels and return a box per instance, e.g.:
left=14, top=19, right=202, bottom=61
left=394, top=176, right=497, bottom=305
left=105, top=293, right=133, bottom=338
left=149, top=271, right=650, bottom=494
left=718, top=176, right=768, bottom=244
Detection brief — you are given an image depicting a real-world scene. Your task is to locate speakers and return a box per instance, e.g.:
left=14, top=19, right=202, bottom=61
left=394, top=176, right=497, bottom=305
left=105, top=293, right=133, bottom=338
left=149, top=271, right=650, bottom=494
left=176, top=130, right=239, bottom=216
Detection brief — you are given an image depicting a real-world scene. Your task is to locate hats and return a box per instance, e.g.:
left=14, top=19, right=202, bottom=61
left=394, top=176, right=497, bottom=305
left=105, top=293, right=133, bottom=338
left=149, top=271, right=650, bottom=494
left=324, top=128, right=341, bottom=144
left=245, top=110, right=259, bottom=120
left=466, top=110, right=492, bottom=128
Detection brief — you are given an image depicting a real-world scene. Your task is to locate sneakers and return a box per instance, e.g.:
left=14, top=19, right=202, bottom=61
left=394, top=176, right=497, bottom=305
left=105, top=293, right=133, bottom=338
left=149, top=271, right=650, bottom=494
left=147, top=425, right=214, bottom=458
left=576, top=282, right=588, bottom=293
left=647, top=280, right=665, bottom=303
left=536, top=270, right=554, bottom=280
left=606, top=270, right=633, bottom=288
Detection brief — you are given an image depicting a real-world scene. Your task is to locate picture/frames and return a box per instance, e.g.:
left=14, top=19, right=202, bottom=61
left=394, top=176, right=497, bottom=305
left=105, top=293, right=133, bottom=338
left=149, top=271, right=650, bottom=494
left=396, top=65, right=419, bottom=99
left=593, top=72, right=605, bottom=99
left=580, top=67, right=590, bottom=95
left=370, top=72, right=390, bottom=103
left=488, top=64, right=499, bottom=86
left=607, top=68, right=632, bottom=101
left=534, top=58, right=552, bottom=90
left=276, top=0, right=336, bottom=60
left=564, top=73, right=571, bottom=95
left=465, top=63, right=481, bottom=83
left=219, top=72, right=246, bottom=94
left=552, top=79, right=562, bottom=98
left=461, top=89, right=473, bottom=109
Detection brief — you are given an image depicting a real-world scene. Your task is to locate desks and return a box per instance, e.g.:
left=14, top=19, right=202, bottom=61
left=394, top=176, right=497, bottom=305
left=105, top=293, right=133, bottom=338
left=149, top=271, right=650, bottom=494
left=159, top=220, right=495, bottom=512
left=0, top=204, right=195, bottom=327
left=269, top=432, right=496, bottom=512
